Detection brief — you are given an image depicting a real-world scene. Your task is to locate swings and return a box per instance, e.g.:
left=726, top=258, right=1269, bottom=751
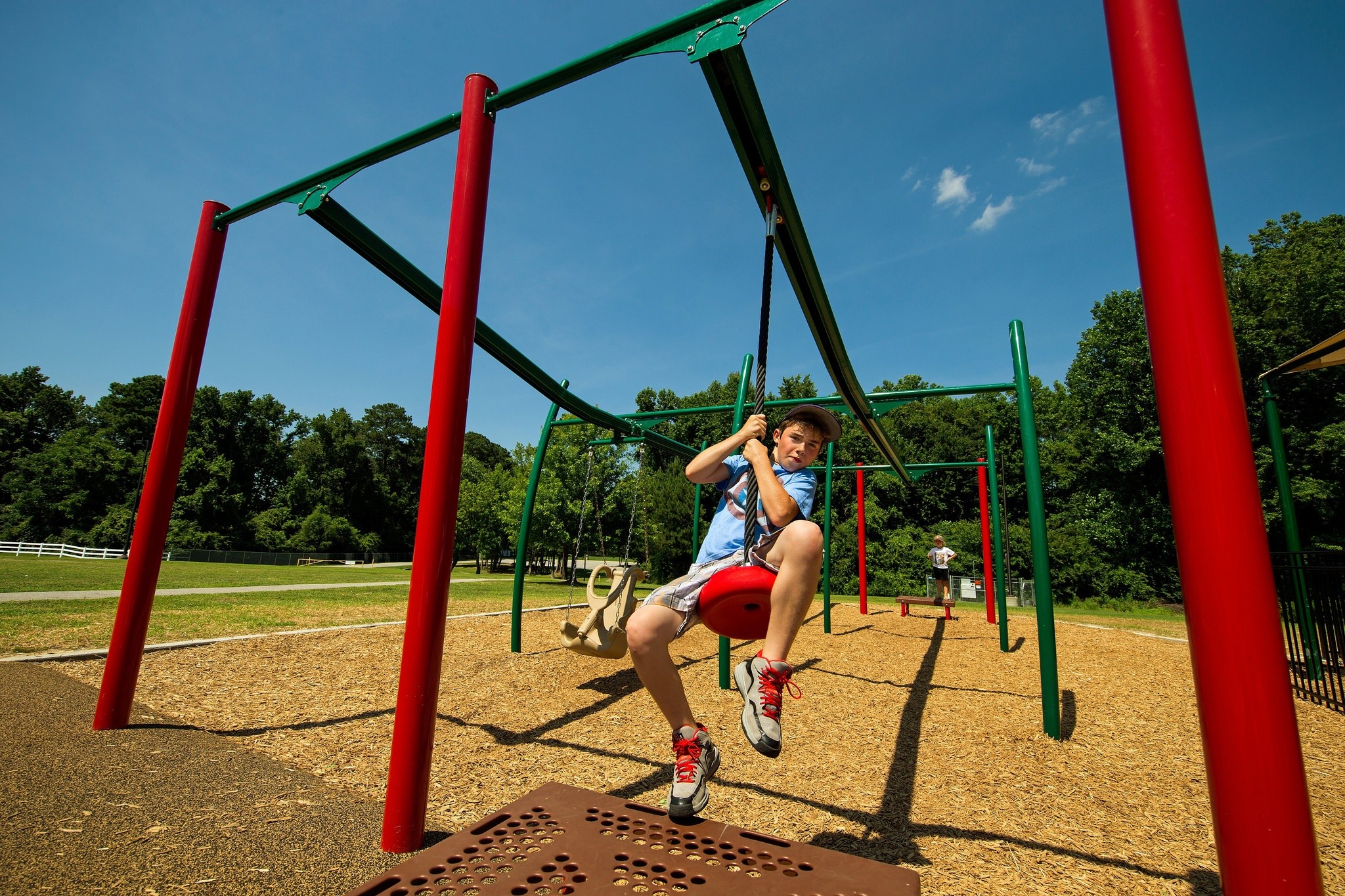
left=561, top=439, right=650, bottom=660
left=698, top=199, right=780, bottom=640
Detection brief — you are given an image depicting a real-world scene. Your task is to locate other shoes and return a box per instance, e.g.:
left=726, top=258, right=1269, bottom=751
left=934, top=596, right=942, bottom=600
left=943, top=598, right=949, bottom=601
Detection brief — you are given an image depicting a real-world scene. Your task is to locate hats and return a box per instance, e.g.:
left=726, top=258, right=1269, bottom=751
left=934, top=535, right=944, bottom=541
left=779, top=404, right=842, bottom=442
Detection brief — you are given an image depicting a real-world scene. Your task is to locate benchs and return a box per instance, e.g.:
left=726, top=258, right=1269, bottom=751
left=896, top=595, right=955, bottom=620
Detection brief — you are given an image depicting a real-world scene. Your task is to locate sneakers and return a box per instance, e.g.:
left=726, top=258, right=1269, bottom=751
left=667, top=723, right=720, bottom=817
left=735, top=649, right=801, bottom=759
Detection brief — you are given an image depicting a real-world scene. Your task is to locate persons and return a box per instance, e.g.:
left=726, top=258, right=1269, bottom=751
left=627, top=402, right=842, bottom=818
left=927, top=535, right=957, bottom=600
